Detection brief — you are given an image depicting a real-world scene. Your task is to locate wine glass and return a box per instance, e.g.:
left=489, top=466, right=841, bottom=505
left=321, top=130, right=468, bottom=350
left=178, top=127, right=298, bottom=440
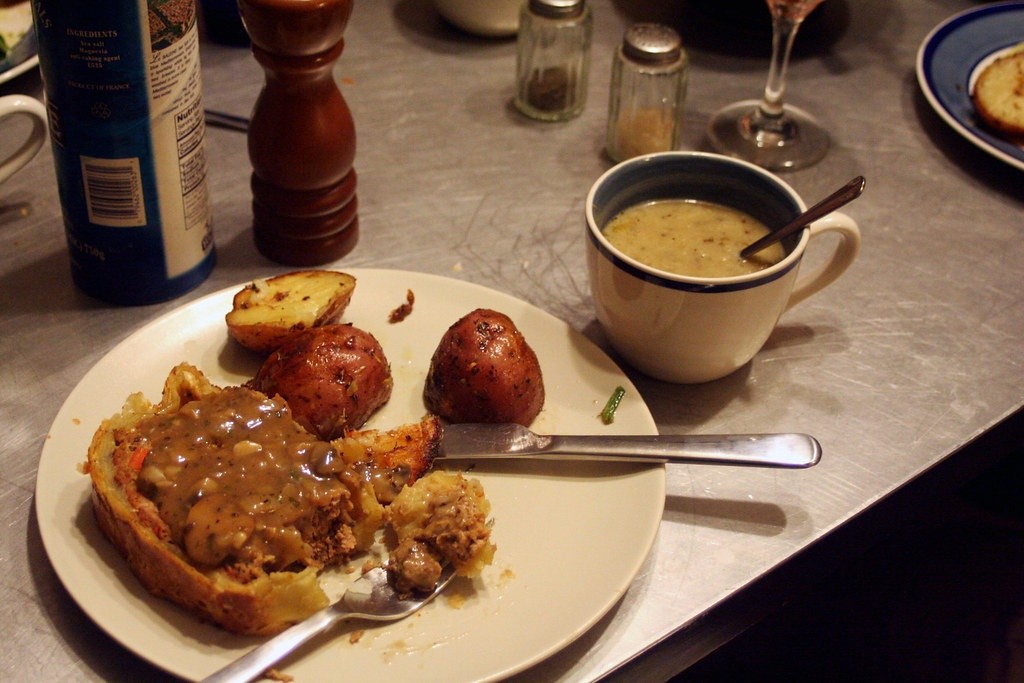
left=705, top=0, right=832, bottom=170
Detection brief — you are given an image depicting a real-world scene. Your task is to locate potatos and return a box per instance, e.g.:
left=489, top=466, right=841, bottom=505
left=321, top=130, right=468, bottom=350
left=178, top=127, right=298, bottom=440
left=423, top=308, right=546, bottom=429
left=974, top=49, right=1024, bottom=133
left=225, top=269, right=358, bottom=354
left=247, top=323, right=393, bottom=442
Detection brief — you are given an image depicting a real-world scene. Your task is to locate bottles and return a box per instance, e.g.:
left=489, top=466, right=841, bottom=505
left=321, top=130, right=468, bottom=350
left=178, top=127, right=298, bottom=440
left=513, top=0, right=593, bottom=121
left=606, top=22, right=691, bottom=164
left=29, top=0, right=217, bottom=305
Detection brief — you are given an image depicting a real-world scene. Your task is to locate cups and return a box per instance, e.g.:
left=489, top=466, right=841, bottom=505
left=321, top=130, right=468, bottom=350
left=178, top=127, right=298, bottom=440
left=434, top=0, right=524, bottom=36
left=585, top=149, right=859, bottom=384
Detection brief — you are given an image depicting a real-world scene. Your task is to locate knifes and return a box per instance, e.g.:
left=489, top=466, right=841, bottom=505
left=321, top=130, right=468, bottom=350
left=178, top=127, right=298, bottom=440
left=434, top=423, right=822, bottom=469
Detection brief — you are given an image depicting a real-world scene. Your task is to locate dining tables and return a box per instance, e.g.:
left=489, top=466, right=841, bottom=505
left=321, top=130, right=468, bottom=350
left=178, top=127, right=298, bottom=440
left=0, top=0, right=1024, bottom=683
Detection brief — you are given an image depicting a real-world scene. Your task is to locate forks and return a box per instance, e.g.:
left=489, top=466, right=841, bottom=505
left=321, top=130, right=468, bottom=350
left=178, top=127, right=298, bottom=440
left=197, top=517, right=495, bottom=683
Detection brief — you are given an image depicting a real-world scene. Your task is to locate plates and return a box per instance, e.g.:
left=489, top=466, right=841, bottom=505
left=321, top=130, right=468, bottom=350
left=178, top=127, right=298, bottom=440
left=34, top=269, right=666, bottom=683
left=0, top=0, right=39, bottom=83
left=915, top=0, right=1024, bottom=170
